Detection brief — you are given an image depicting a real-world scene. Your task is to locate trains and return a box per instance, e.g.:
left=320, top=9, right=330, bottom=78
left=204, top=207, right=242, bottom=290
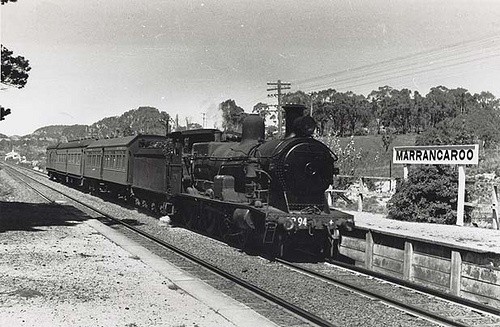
left=47, top=104, right=355, bottom=261
